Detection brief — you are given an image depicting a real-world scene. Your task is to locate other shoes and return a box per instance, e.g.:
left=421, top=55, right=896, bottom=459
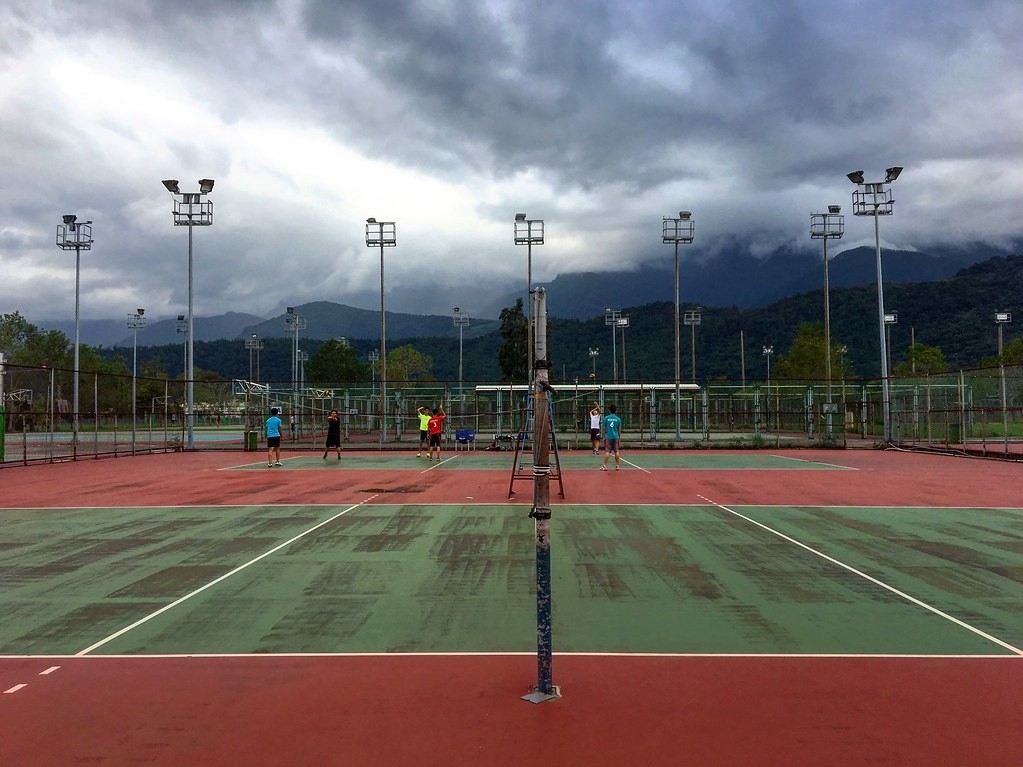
left=338, top=455, right=341, bottom=459
left=593, top=449, right=599, bottom=455
left=600, top=464, right=608, bottom=471
left=276, top=462, right=282, bottom=466
left=437, top=458, right=442, bottom=462
left=430, top=458, right=433, bottom=461
left=426, top=452, right=430, bottom=457
left=268, top=463, right=272, bottom=467
left=615, top=465, right=619, bottom=470
left=323, top=455, right=327, bottom=458
left=417, top=453, right=421, bottom=457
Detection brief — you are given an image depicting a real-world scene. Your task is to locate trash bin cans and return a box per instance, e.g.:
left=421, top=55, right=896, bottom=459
left=949, top=423, right=960, bottom=440
left=244, top=431, right=257, bottom=450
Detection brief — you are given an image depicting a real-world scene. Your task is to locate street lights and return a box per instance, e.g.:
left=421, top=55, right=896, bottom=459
left=683, top=307, right=703, bottom=431
left=588, top=347, right=600, bottom=401
left=364, top=217, right=398, bottom=448
left=617, top=312, right=631, bottom=427
left=175, top=314, right=189, bottom=429
left=762, top=345, right=775, bottom=428
left=993, top=312, right=1013, bottom=458
left=886, top=314, right=898, bottom=441
left=161, top=178, right=215, bottom=450
left=661, top=210, right=695, bottom=439
left=283, top=305, right=309, bottom=440
left=452, top=307, right=470, bottom=430
left=54, top=214, right=97, bottom=447
left=245, top=334, right=265, bottom=430
left=836, top=345, right=848, bottom=406
left=367, top=349, right=379, bottom=415
left=604, top=307, right=622, bottom=415
left=513, top=212, right=546, bottom=433
left=846, top=164, right=898, bottom=447
left=126, top=308, right=147, bottom=455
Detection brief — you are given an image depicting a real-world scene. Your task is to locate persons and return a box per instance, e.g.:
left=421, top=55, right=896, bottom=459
left=417, top=404, right=447, bottom=461
left=590, top=402, right=603, bottom=455
left=266, top=408, right=283, bottom=466
left=600, top=405, right=622, bottom=470
left=323, top=410, right=342, bottom=460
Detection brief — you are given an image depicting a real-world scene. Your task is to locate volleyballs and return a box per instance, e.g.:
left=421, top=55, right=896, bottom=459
left=590, top=374, right=595, bottom=379
left=323, top=390, right=329, bottom=396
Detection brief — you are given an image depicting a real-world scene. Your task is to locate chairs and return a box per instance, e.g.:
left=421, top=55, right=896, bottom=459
left=455, top=430, right=476, bottom=452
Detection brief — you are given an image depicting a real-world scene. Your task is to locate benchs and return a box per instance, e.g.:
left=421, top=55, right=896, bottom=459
left=493, top=438, right=554, bottom=452
